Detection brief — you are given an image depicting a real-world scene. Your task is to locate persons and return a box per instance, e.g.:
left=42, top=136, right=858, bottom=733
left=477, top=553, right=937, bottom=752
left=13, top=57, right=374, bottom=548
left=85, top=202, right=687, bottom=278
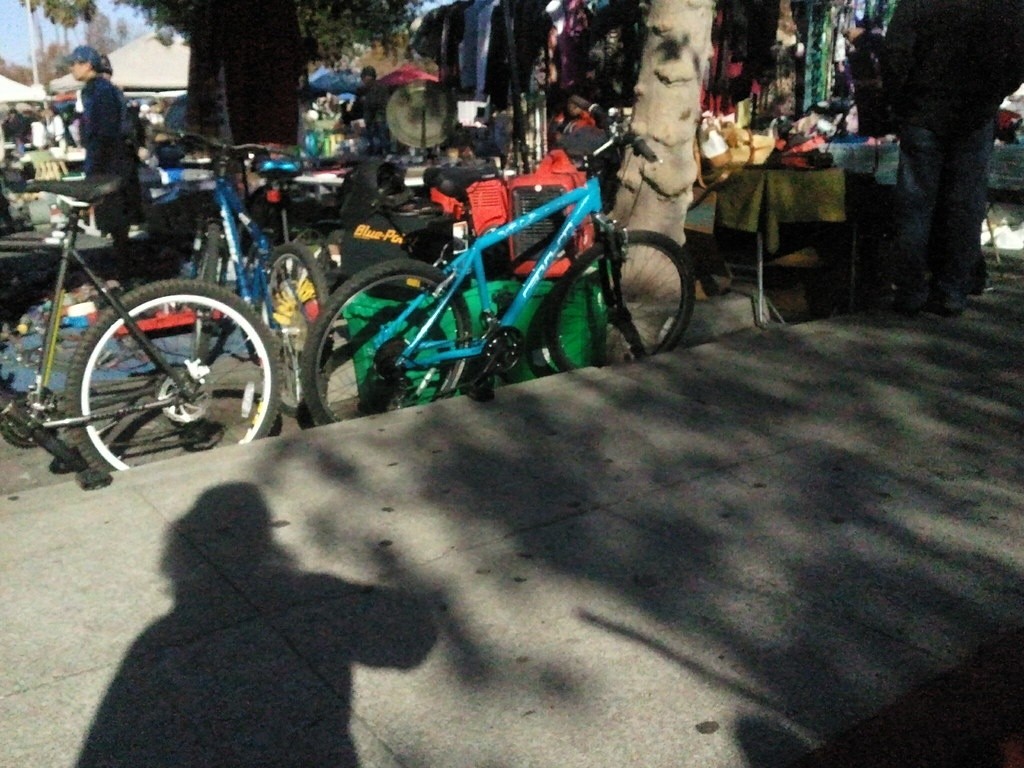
left=3, top=44, right=143, bottom=280
left=848, top=0, right=1024, bottom=315
left=306, top=66, right=392, bottom=154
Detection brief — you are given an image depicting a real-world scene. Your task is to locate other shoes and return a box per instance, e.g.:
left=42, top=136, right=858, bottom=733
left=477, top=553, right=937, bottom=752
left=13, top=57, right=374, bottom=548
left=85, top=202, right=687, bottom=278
left=934, top=272, right=984, bottom=294
left=865, top=286, right=922, bottom=314
left=88, top=253, right=116, bottom=265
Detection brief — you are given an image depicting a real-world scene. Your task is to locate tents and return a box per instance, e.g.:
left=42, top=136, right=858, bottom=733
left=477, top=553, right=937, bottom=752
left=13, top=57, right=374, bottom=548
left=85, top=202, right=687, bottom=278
left=0, top=74, right=45, bottom=102
left=48, top=24, right=191, bottom=90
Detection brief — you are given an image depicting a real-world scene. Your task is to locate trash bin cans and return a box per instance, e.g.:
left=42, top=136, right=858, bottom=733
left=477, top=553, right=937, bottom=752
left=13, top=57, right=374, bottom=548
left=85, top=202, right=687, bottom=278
left=312, top=120, right=336, bottom=159
left=342, top=277, right=518, bottom=416
left=507, top=260, right=614, bottom=383
left=19, top=150, right=61, bottom=225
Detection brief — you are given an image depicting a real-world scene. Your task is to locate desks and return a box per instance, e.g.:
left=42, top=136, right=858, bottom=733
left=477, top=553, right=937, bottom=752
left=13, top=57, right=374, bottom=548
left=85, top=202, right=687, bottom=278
left=298, top=164, right=517, bottom=187
left=707, top=140, right=1024, bottom=327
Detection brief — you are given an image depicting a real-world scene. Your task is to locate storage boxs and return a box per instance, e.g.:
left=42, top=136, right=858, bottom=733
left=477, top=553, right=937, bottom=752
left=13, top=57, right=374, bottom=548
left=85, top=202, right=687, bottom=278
left=341, top=255, right=616, bottom=417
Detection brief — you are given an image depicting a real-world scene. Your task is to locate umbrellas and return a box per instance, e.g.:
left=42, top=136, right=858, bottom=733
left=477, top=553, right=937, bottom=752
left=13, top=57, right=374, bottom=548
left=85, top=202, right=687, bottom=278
left=380, top=64, right=439, bottom=89
left=299, top=66, right=364, bottom=94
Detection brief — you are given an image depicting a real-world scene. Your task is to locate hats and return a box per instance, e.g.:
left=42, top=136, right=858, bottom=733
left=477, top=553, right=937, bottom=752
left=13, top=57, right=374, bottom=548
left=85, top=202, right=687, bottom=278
left=361, top=65, right=376, bottom=77
left=63, top=46, right=101, bottom=66
left=98, top=54, right=112, bottom=74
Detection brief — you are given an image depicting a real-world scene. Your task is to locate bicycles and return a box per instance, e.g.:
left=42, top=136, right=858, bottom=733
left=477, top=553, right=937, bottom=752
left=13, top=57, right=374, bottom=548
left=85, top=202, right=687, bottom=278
left=0, top=174, right=285, bottom=490
left=151, top=125, right=333, bottom=424
left=296, top=95, right=697, bottom=427
